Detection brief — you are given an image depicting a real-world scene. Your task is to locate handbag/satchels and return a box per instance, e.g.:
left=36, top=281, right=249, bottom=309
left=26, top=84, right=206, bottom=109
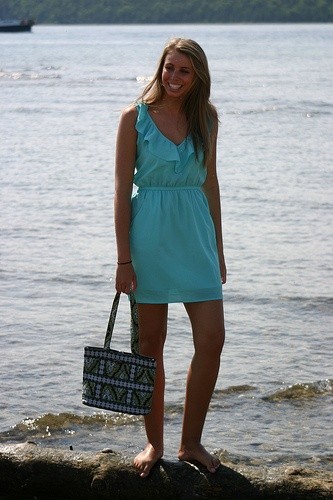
left=82, top=288, right=157, bottom=415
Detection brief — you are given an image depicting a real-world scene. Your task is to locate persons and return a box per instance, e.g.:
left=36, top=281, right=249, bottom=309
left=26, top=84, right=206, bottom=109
left=114, top=39, right=227, bottom=476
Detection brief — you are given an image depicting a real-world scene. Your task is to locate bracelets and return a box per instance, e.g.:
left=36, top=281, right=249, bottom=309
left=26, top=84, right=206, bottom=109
left=116, top=260, right=133, bottom=265
